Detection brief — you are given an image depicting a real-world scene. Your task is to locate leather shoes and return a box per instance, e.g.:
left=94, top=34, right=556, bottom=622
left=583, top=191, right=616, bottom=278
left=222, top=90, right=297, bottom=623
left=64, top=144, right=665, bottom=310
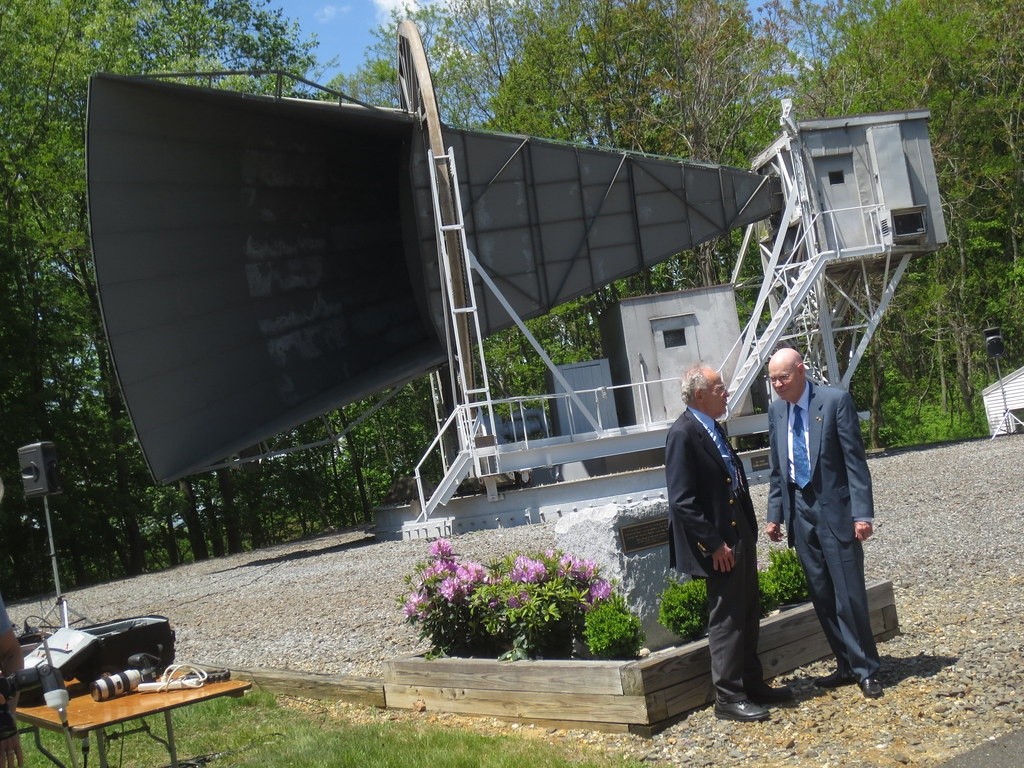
left=714, top=699, right=770, bottom=721
left=813, top=667, right=856, bottom=688
left=747, top=680, right=793, bottom=705
left=859, top=674, right=883, bottom=699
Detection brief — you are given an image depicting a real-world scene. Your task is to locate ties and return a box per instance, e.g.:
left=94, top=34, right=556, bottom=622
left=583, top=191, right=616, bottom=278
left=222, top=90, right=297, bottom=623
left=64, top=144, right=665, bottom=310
left=793, top=404, right=811, bottom=489
left=714, top=419, right=745, bottom=493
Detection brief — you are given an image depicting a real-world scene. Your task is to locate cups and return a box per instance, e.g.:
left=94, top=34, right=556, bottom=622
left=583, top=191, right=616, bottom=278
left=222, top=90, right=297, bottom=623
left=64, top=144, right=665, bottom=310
left=89, top=669, right=141, bottom=702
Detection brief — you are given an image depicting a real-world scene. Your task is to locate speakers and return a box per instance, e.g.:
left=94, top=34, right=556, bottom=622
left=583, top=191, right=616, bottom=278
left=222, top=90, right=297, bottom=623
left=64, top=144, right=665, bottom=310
left=17, top=441, right=63, bottom=499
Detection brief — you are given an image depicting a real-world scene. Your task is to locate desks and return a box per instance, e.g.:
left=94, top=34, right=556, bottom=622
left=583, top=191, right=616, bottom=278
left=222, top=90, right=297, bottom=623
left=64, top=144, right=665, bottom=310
left=17, top=671, right=254, bottom=768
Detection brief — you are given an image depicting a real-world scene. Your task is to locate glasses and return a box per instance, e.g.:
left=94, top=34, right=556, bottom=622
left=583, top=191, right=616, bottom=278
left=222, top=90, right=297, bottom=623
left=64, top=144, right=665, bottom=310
left=769, top=363, right=799, bottom=384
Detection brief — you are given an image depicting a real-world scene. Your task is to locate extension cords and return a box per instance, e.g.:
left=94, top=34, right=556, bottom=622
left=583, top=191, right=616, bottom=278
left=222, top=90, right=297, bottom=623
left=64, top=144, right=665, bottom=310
left=137, top=678, right=201, bottom=693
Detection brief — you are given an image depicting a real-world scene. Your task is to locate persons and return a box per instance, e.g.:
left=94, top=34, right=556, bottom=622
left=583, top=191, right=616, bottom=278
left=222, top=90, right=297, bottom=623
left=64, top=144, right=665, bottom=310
left=0, top=477, right=25, bottom=768
left=665, top=363, right=791, bottom=720
left=764, top=346, right=883, bottom=698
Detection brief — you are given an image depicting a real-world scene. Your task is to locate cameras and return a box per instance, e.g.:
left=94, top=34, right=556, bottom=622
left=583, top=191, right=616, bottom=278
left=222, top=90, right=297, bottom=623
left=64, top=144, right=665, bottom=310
left=90, top=653, right=157, bottom=702
left=0, top=664, right=68, bottom=741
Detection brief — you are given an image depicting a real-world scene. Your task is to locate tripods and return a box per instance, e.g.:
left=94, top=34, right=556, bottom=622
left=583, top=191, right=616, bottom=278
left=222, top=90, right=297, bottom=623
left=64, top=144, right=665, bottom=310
left=35, top=496, right=96, bottom=632
